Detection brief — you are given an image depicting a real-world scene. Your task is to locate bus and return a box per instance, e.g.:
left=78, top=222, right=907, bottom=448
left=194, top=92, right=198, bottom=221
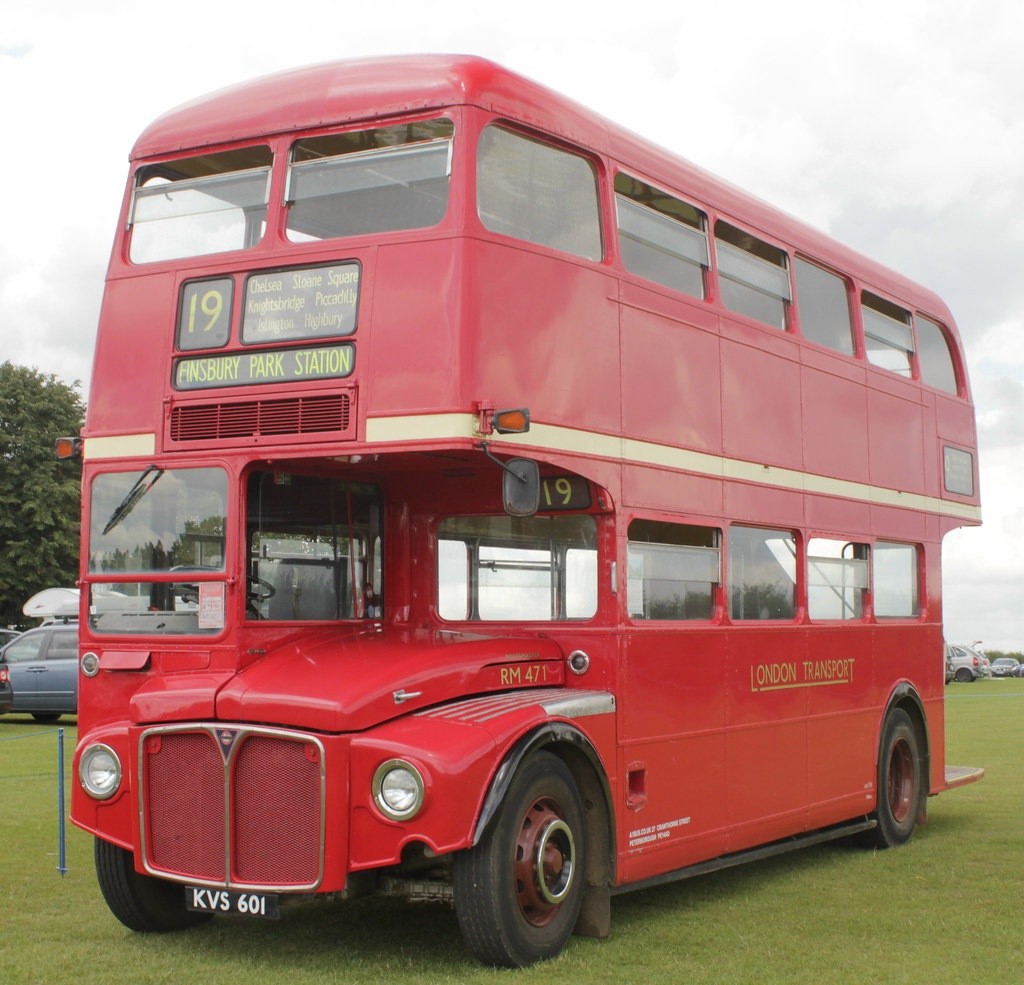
left=944, top=643, right=955, bottom=685
left=55, top=50, right=987, bottom=975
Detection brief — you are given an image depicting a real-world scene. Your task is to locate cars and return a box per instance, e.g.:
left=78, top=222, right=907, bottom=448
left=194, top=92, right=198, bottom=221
left=991, top=657, right=1019, bottom=677
left=1017, top=663, right=1024, bottom=677
left=0, top=652, right=14, bottom=719
left=0, top=628, right=51, bottom=667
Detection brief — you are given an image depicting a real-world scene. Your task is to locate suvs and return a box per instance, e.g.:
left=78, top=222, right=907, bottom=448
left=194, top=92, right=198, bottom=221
left=0, top=587, right=130, bottom=723
left=978, top=652, right=991, bottom=681
left=951, top=645, right=983, bottom=682
